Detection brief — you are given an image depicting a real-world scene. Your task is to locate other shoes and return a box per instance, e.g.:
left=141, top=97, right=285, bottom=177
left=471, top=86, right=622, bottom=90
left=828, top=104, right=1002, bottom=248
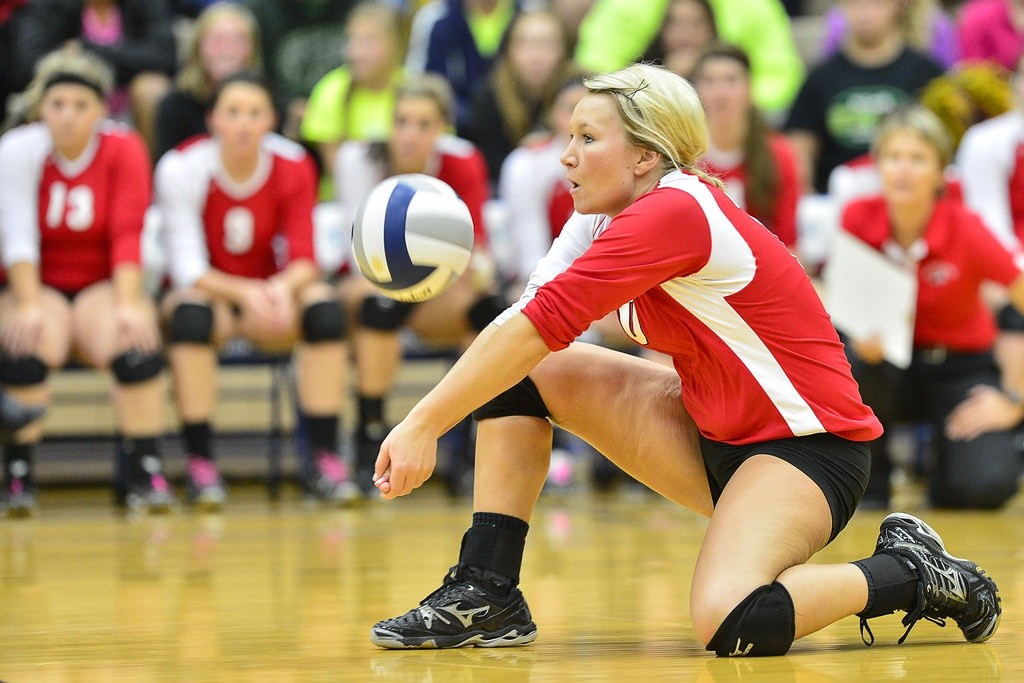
left=118, top=456, right=169, bottom=512
left=357, top=452, right=387, bottom=499
left=298, top=444, right=365, bottom=506
left=182, top=449, right=227, bottom=506
left=1, top=458, right=40, bottom=514
left=446, top=443, right=479, bottom=493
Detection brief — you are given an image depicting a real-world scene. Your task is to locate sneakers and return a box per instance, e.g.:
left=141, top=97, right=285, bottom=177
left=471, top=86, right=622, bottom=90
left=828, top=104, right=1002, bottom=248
left=369, top=561, right=537, bottom=646
left=872, top=512, right=1002, bottom=645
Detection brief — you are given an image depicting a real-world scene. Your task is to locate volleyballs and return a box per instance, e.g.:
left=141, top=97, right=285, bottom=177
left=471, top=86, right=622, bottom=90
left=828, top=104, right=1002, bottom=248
left=351, top=172, right=476, bottom=304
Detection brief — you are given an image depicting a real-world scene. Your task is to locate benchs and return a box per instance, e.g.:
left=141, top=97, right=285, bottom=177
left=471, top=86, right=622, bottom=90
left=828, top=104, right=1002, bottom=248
left=4, top=336, right=653, bottom=501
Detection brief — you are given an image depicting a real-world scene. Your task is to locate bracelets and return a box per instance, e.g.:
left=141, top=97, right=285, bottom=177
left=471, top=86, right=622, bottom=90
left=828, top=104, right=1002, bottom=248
left=1004, top=386, right=1024, bottom=407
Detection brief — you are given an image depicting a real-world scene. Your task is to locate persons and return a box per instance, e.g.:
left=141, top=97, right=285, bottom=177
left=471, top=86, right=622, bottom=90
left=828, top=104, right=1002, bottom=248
left=372, top=59, right=1001, bottom=657
left=500, top=69, right=655, bottom=499
left=332, top=76, right=509, bottom=496
left=0, top=0, right=1024, bottom=264
left=692, top=46, right=799, bottom=253
left=826, top=108, right=1024, bottom=513
left=959, top=104, right=1024, bottom=409
left=152, top=71, right=362, bottom=507
left=0, top=49, right=171, bottom=520
